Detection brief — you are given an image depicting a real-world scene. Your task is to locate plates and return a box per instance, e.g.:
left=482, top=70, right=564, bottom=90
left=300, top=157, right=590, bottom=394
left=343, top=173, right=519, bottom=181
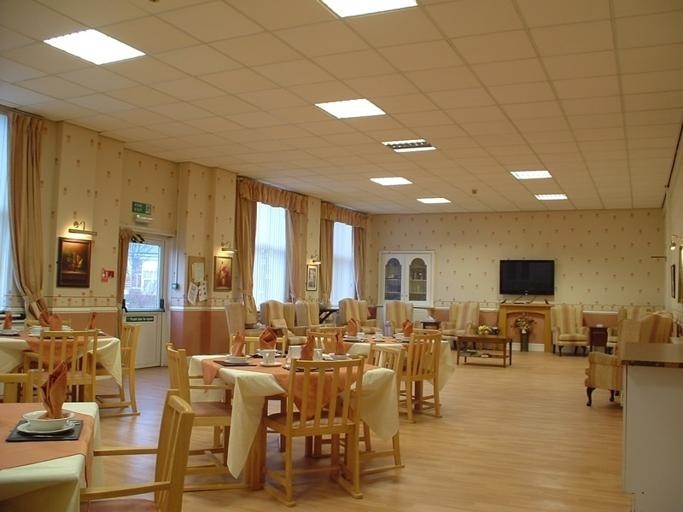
left=28, top=333, right=41, bottom=337
left=1, top=332, right=18, bottom=335
left=260, top=362, right=283, bottom=367
left=224, top=360, right=249, bottom=364
left=342, top=338, right=360, bottom=342
left=16, top=422, right=75, bottom=435
left=373, top=339, right=385, bottom=342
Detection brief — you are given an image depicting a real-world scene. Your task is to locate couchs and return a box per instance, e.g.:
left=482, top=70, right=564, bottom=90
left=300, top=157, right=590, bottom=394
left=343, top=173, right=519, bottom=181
left=583, top=312, right=674, bottom=407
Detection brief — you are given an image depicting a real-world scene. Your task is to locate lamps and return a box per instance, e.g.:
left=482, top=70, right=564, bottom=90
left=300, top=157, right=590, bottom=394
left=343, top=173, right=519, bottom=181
left=668, top=230, right=683, bottom=253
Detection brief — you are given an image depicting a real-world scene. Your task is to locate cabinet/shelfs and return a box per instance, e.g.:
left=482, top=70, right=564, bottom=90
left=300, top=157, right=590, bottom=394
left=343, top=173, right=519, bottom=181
left=375, top=250, right=436, bottom=330
left=619, top=342, right=683, bottom=511
left=497, top=303, right=555, bottom=353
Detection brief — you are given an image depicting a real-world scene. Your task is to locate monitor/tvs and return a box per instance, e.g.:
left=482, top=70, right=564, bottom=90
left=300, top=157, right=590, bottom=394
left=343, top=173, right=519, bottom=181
left=499, top=259, right=555, bottom=296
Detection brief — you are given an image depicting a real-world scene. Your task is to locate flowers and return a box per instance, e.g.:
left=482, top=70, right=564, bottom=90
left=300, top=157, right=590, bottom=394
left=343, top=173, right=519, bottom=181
left=510, top=312, right=539, bottom=334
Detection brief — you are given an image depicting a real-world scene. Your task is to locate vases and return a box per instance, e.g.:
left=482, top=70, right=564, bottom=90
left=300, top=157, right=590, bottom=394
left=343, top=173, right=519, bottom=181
left=520, top=333, right=529, bottom=353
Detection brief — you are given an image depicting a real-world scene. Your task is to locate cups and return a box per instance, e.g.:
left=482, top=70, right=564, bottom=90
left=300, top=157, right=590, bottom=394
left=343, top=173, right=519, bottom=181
left=289, top=346, right=301, bottom=360
left=263, top=350, right=276, bottom=363
left=314, top=349, right=322, bottom=360
left=377, top=334, right=383, bottom=339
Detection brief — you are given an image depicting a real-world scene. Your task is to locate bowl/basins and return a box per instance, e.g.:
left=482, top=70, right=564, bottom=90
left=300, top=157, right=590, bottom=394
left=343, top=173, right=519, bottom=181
left=401, top=336, right=411, bottom=342
left=22, top=410, right=74, bottom=430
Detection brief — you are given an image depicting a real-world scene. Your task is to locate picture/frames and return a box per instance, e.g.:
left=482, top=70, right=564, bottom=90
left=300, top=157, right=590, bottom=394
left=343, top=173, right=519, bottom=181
left=670, top=264, right=676, bottom=300
left=305, top=263, right=319, bottom=292
left=56, top=236, right=93, bottom=290
left=212, top=255, right=234, bottom=293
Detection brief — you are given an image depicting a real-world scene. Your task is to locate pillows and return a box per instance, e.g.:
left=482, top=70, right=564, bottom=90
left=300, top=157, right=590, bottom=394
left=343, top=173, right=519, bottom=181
left=269, top=318, right=297, bottom=338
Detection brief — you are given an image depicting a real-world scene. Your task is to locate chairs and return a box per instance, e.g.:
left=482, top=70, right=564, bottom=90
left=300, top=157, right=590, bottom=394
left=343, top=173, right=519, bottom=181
left=294, top=299, right=320, bottom=336
left=0, top=336, right=123, bottom=402
left=385, top=299, right=414, bottom=337
left=282, top=302, right=298, bottom=334
left=607, top=304, right=642, bottom=356
left=337, top=341, right=408, bottom=481
left=95, top=322, right=141, bottom=419
left=231, top=332, right=288, bottom=359
left=437, top=300, right=482, bottom=352
left=549, top=303, right=591, bottom=358
left=78, top=393, right=196, bottom=511
left=338, top=298, right=383, bottom=335
left=608, top=303, right=628, bottom=342
left=22, top=327, right=99, bottom=403
left=165, top=342, right=250, bottom=493
left=0, top=368, right=35, bottom=403
left=261, top=299, right=308, bottom=357
left=309, top=301, right=320, bottom=326
left=222, top=301, right=289, bottom=360
left=398, top=331, right=443, bottom=424
left=260, top=354, right=366, bottom=508
left=305, top=330, right=341, bottom=356
